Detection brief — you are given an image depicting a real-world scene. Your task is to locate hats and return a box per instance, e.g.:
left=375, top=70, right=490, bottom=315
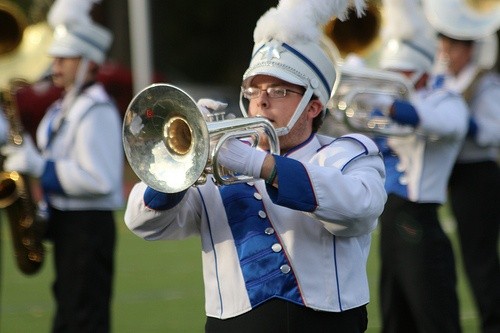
left=423, top=0, right=500, bottom=44
left=379, top=0, right=440, bottom=75
left=241, top=0, right=369, bottom=124
left=45, top=0, right=114, bottom=68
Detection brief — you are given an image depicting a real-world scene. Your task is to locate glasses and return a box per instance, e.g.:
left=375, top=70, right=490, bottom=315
left=242, top=85, right=303, bottom=99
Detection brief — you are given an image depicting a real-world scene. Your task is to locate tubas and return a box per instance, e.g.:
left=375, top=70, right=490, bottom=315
left=122, top=84, right=280, bottom=194
left=329, top=66, right=418, bottom=137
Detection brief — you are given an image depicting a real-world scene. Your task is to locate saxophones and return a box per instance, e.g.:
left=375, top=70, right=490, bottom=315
left=0, top=93, right=46, bottom=277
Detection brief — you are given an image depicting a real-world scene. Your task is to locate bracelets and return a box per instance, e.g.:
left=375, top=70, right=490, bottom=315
left=267, top=165, right=277, bottom=186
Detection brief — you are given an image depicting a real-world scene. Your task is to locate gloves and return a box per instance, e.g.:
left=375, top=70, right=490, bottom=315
left=196, top=98, right=234, bottom=126
left=0, top=132, right=47, bottom=179
left=357, top=92, right=396, bottom=115
left=204, top=133, right=267, bottom=182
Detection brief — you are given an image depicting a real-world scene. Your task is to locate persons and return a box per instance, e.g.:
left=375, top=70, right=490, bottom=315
left=0, top=0, right=500, bottom=333
